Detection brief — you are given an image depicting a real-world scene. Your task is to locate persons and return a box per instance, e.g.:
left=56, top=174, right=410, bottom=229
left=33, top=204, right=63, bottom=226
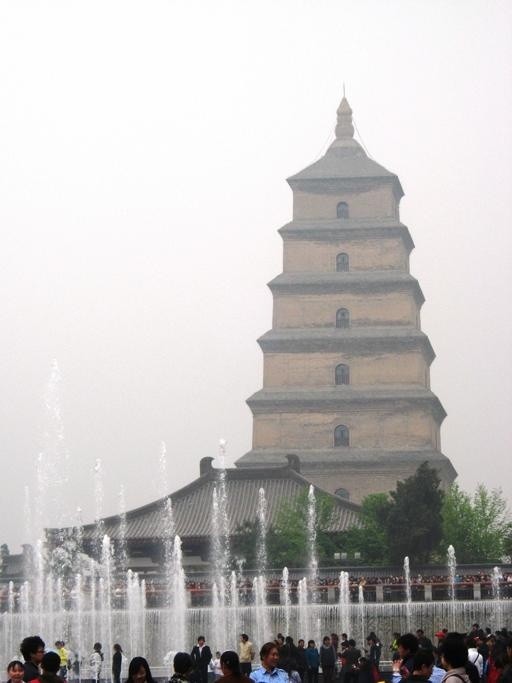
left=7, top=622, right=512, bottom=682
left=148, top=569, right=512, bottom=593
